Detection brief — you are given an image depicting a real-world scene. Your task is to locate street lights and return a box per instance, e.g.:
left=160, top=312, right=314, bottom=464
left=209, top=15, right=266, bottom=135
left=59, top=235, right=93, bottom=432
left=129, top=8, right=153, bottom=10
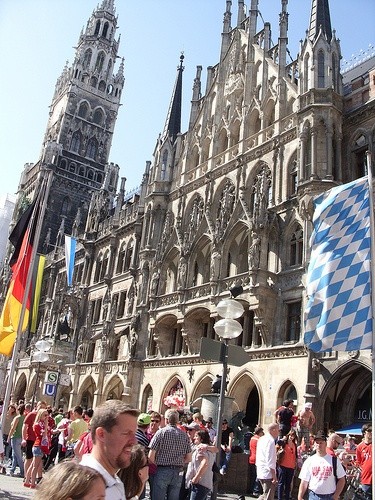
left=29, top=339, right=52, bottom=412
left=211, top=299, right=245, bottom=499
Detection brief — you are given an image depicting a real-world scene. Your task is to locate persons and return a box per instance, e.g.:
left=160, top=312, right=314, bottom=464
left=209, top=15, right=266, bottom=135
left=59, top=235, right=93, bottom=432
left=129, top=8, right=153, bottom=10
left=256, top=423, right=279, bottom=500
left=33, top=461, right=106, bottom=500
left=129, top=412, right=151, bottom=500
left=297, top=434, right=346, bottom=500
left=186, top=430, right=217, bottom=500
left=353, top=423, right=372, bottom=500
left=77, top=398, right=140, bottom=500
left=0, top=386, right=372, bottom=487
left=148, top=409, right=192, bottom=500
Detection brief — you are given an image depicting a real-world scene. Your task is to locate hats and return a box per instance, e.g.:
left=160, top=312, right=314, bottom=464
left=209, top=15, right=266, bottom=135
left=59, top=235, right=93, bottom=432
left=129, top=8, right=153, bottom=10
left=137, top=413, right=152, bottom=425
left=221, top=418, right=228, bottom=424
left=185, top=422, right=199, bottom=430
left=313, top=434, right=327, bottom=441
left=205, top=417, right=214, bottom=424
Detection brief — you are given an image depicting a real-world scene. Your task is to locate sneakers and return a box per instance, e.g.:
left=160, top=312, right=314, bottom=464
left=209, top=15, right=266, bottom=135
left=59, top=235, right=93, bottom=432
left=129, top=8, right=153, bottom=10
left=23, top=482, right=31, bottom=487
left=37, top=477, right=42, bottom=483
left=29, top=483, right=40, bottom=489
left=0, top=452, right=51, bottom=477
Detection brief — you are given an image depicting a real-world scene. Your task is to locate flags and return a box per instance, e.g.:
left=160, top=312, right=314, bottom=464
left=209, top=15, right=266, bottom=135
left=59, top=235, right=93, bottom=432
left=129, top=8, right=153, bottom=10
left=0, top=192, right=42, bottom=359
left=300, top=175, right=374, bottom=351
left=64, top=234, right=76, bottom=288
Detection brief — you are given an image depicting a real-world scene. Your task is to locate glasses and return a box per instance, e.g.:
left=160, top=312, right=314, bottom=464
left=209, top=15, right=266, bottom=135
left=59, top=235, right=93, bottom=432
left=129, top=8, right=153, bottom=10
left=334, top=440, right=340, bottom=445
left=365, top=429, right=372, bottom=432
left=140, top=456, right=149, bottom=468
left=222, top=422, right=228, bottom=425
left=150, top=420, right=160, bottom=423
left=206, top=421, right=210, bottom=424
